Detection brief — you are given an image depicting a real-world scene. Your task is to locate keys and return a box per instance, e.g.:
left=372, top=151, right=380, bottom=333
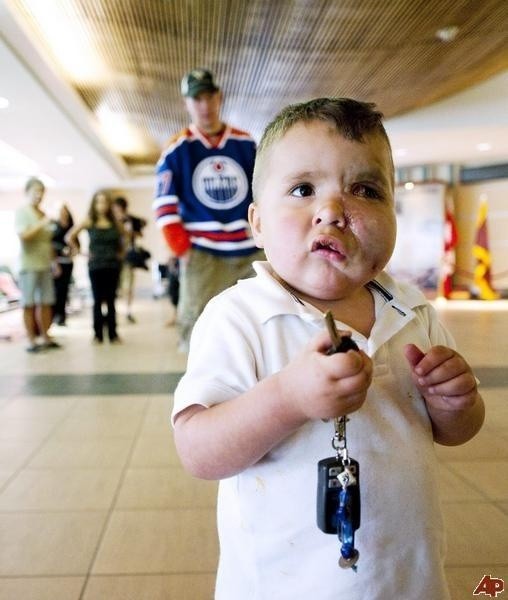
left=316, top=309, right=362, bottom=533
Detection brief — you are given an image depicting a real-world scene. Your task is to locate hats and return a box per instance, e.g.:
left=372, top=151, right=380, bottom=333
left=180, top=69, right=218, bottom=97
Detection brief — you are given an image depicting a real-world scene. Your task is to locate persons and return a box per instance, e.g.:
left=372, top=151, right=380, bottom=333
left=152, top=66, right=267, bottom=357
left=12, top=171, right=146, bottom=353
left=171, top=97, right=485, bottom=599
left=164, top=256, right=180, bottom=328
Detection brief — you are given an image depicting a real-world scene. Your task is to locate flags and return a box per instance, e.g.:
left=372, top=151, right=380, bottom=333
left=469, top=202, right=501, bottom=301
left=436, top=205, right=460, bottom=300
